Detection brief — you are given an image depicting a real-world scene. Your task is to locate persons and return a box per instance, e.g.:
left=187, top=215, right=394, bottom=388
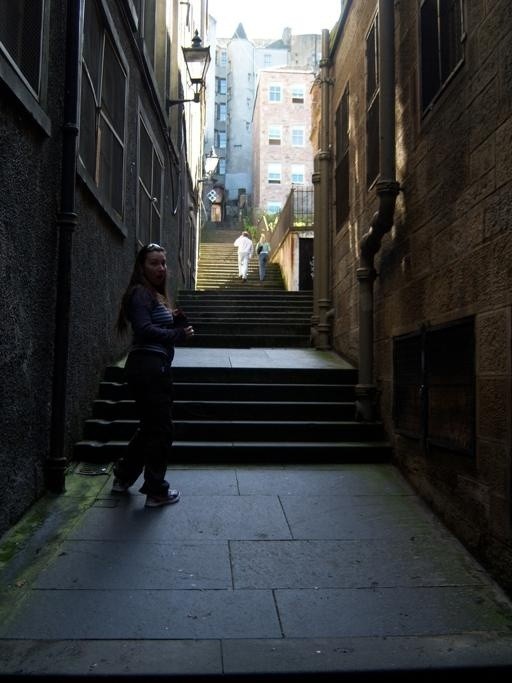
left=233, top=231, right=271, bottom=282
left=111, top=242, right=198, bottom=508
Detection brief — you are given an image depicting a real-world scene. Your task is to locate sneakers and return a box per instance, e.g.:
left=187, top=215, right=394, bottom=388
left=110, top=478, right=130, bottom=495
left=144, top=489, right=180, bottom=507
left=239, top=276, right=247, bottom=282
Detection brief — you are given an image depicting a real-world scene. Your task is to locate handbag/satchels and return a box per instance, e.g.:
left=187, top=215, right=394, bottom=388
left=257, top=246, right=263, bottom=254
left=138, top=367, right=175, bottom=405
